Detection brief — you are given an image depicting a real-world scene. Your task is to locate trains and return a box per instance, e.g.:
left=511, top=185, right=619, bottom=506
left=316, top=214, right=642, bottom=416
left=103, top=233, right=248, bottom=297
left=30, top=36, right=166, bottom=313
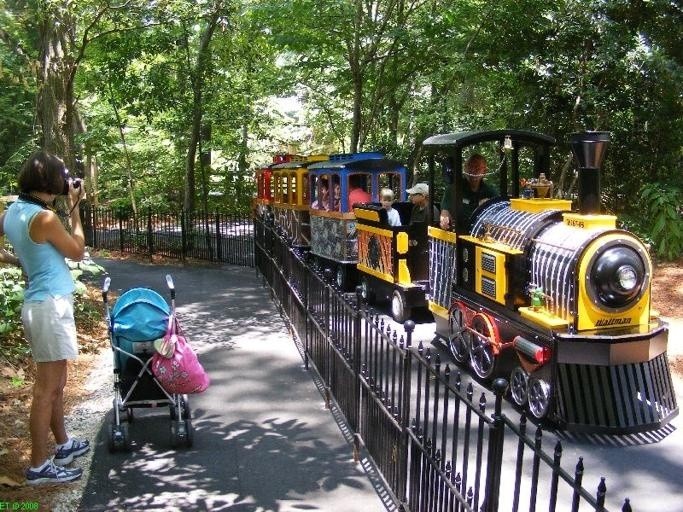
left=252, top=126, right=680, bottom=437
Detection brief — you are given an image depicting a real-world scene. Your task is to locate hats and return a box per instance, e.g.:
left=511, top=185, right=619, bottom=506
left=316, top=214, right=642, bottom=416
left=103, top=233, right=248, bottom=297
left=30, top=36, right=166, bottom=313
left=403, top=182, right=430, bottom=196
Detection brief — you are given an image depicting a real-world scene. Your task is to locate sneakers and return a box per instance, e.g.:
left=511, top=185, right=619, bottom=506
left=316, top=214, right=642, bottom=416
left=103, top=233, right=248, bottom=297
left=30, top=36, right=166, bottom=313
left=51, top=437, right=90, bottom=466
left=23, top=454, right=83, bottom=486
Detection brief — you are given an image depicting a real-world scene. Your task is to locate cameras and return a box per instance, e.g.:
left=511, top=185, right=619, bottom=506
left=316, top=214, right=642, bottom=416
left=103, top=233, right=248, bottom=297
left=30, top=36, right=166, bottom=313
left=61, top=176, right=81, bottom=196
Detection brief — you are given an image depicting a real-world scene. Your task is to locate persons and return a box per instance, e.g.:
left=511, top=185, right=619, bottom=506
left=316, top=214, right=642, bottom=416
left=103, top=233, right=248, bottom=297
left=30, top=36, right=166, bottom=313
left=405, top=183, right=441, bottom=227
left=439, top=154, right=502, bottom=235
left=311, top=174, right=372, bottom=212
left=0, top=150, right=91, bottom=485
left=379, top=188, right=401, bottom=226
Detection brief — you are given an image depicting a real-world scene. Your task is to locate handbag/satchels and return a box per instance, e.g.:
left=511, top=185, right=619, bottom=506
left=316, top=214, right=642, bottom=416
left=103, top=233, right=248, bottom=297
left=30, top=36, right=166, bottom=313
left=150, top=313, right=212, bottom=396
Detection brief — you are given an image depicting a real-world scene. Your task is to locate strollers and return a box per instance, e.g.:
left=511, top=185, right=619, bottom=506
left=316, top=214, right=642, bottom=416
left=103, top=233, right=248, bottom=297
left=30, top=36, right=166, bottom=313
left=100, top=274, right=196, bottom=453
